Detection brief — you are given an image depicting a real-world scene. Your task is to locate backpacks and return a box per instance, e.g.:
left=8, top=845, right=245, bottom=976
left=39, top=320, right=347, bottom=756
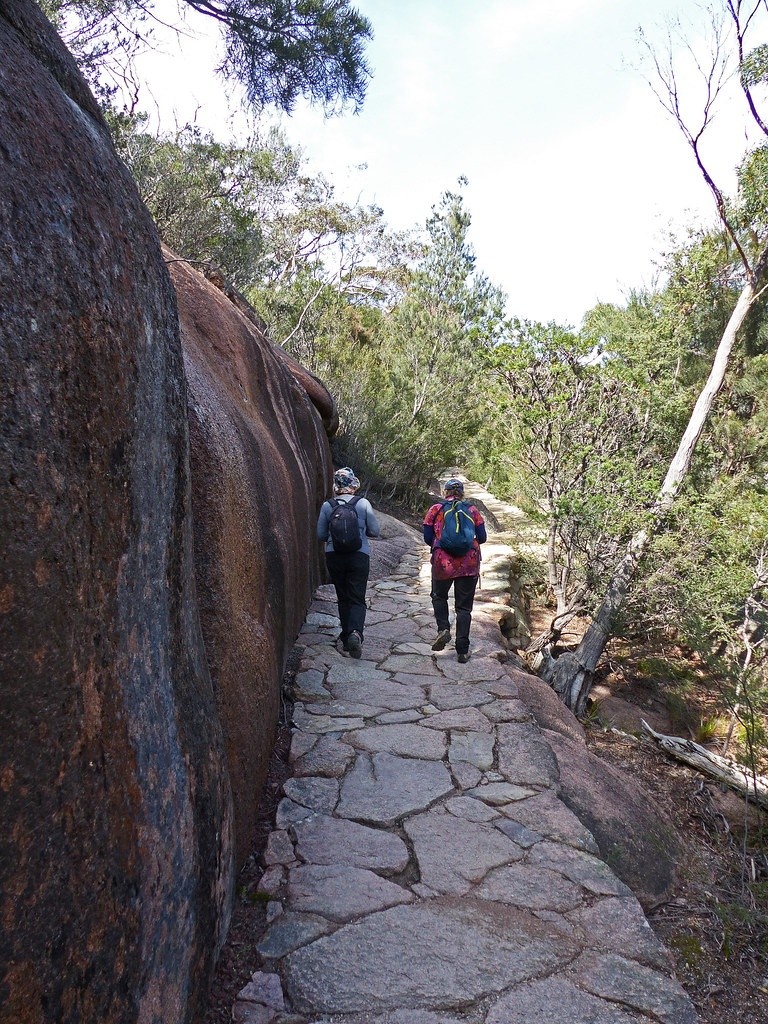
left=437, top=500, right=475, bottom=557
left=327, top=496, right=363, bottom=553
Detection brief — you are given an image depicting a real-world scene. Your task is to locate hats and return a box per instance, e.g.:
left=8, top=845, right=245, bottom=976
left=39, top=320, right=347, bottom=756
left=444, top=479, right=463, bottom=490
left=333, top=467, right=360, bottom=493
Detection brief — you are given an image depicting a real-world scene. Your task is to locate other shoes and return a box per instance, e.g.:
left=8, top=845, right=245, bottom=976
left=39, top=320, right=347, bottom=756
left=349, top=633, right=362, bottom=659
left=339, top=633, right=349, bottom=651
left=458, top=653, right=471, bottom=663
left=431, top=629, right=451, bottom=651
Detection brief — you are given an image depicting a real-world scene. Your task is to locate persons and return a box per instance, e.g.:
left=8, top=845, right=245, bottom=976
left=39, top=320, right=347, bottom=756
left=422, top=478, right=487, bottom=664
left=316, top=466, right=380, bottom=659
